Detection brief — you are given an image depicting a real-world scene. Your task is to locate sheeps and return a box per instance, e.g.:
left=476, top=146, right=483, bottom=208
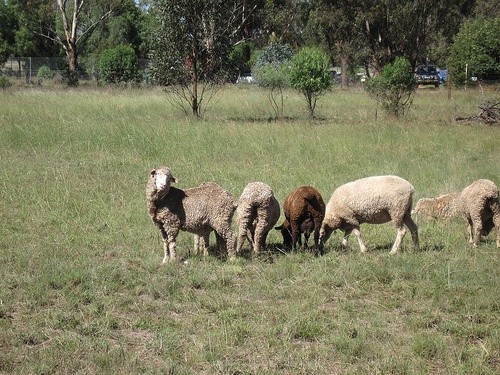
left=274, top=185, right=326, bottom=258
left=235, top=178, right=282, bottom=258
left=144, top=164, right=237, bottom=264
left=318, top=173, right=421, bottom=255
left=410, top=176, right=500, bottom=250
